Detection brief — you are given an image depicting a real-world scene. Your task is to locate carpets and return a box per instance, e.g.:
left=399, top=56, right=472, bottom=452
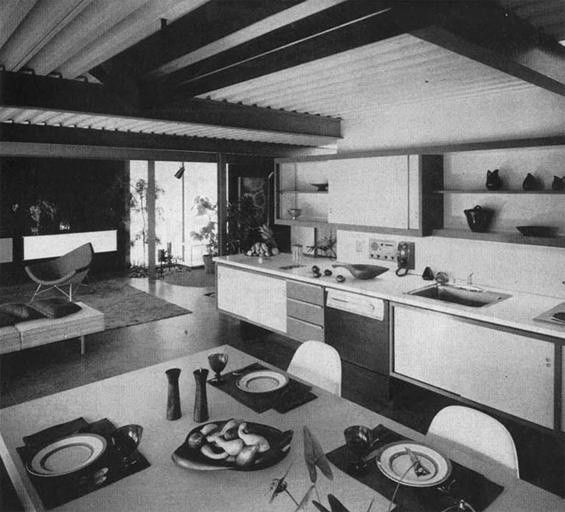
left=154, top=268, right=216, bottom=288
left=0, top=276, right=193, bottom=332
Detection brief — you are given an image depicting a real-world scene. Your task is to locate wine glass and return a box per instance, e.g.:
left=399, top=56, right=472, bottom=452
left=108, top=423, right=143, bottom=465
left=344, top=425, right=379, bottom=467
left=206, top=351, right=230, bottom=383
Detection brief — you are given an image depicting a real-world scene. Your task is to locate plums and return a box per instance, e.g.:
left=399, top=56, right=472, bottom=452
left=337, top=275, right=345, bottom=282
left=324, top=269, right=332, bottom=276
left=313, top=272, right=319, bottom=278
left=312, top=265, right=319, bottom=272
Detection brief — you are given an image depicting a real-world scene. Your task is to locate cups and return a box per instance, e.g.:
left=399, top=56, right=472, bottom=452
left=288, top=209, right=301, bottom=219
left=465, top=209, right=494, bottom=233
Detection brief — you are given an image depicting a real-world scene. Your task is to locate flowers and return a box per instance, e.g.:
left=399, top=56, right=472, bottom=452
left=190, top=194, right=219, bottom=256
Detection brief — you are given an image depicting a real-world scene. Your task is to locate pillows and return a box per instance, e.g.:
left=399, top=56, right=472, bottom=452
left=0, top=303, right=48, bottom=321
left=0, top=309, right=24, bottom=328
left=23, top=298, right=82, bottom=321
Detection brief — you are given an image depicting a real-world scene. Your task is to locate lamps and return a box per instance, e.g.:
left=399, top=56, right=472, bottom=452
left=173, top=166, right=186, bottom=181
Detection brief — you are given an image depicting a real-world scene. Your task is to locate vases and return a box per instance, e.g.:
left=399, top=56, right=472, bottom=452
left=201, top=253, right=216, bottom=273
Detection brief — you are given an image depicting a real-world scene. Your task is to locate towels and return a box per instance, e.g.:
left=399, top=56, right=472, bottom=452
left=21, top=415, right=92, bottom=450
left=270, top=387, right=312, bottom=411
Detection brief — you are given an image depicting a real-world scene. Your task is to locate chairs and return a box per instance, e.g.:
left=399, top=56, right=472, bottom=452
left=287, top=338, right=343, bottom=397
left=424, top=403, right=520, bottom=478
left=23, top=241, right=95, bottom=303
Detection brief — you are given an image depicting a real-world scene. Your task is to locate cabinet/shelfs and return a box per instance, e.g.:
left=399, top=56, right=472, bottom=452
left=272, top=133, right=565, bottom=249
left=216, top=261, right=286, bottom=343
left=287, top=278, right=325, bottom=342
left=388, top=302, right=563, bottom=434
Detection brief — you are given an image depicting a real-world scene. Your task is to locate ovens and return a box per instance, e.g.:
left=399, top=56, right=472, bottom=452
left=324, top=287, right=390, bottom=378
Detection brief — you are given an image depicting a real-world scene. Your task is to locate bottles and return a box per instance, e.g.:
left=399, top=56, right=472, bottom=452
left=164, top=367, right=212, bottom=422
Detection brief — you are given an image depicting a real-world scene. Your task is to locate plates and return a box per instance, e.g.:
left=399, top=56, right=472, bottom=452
left=516, top=225, right=560, bottom=236
left=24, top=432, right=108, bottom=476
left=237, top=369, right=290, bottom=393
left=376, top=441, right=454, bottom=488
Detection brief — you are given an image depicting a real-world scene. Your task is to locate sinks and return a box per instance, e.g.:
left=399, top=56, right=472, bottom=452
left=406, top=283, right=514, bottom=309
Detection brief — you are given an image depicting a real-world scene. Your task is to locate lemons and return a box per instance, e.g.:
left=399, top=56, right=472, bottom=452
left=223, top=428, right=238, bottom=440
left=235, top=443, right=257, bottom=468
left=187, top=430, right=203, bottom=449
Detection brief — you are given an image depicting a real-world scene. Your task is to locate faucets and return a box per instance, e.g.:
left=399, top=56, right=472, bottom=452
left=466, top=272, right=475, bottom=288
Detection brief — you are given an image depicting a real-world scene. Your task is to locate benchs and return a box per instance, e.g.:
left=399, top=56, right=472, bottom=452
left=0, top=300, right=106, bottom=356
